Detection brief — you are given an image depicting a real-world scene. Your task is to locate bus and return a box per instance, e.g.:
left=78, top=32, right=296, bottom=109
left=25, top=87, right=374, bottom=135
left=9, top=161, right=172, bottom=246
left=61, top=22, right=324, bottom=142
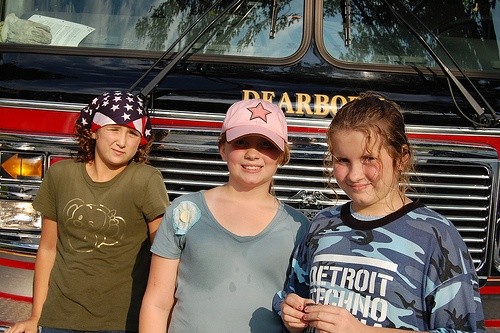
left=1, top=0, right=500, bottom=333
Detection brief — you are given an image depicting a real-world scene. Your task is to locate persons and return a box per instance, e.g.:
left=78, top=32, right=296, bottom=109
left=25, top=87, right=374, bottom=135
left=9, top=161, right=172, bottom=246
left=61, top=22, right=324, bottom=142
left=270, top=89, right=488, bottom=333
left=3, top=89, right=172, bottom=333
left=137, top=98, right=312, bottom=333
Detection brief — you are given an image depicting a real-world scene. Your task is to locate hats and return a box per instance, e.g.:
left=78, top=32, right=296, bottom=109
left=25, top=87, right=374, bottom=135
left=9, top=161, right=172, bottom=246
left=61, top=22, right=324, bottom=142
left=219, top=100, right=290, bottom=152
